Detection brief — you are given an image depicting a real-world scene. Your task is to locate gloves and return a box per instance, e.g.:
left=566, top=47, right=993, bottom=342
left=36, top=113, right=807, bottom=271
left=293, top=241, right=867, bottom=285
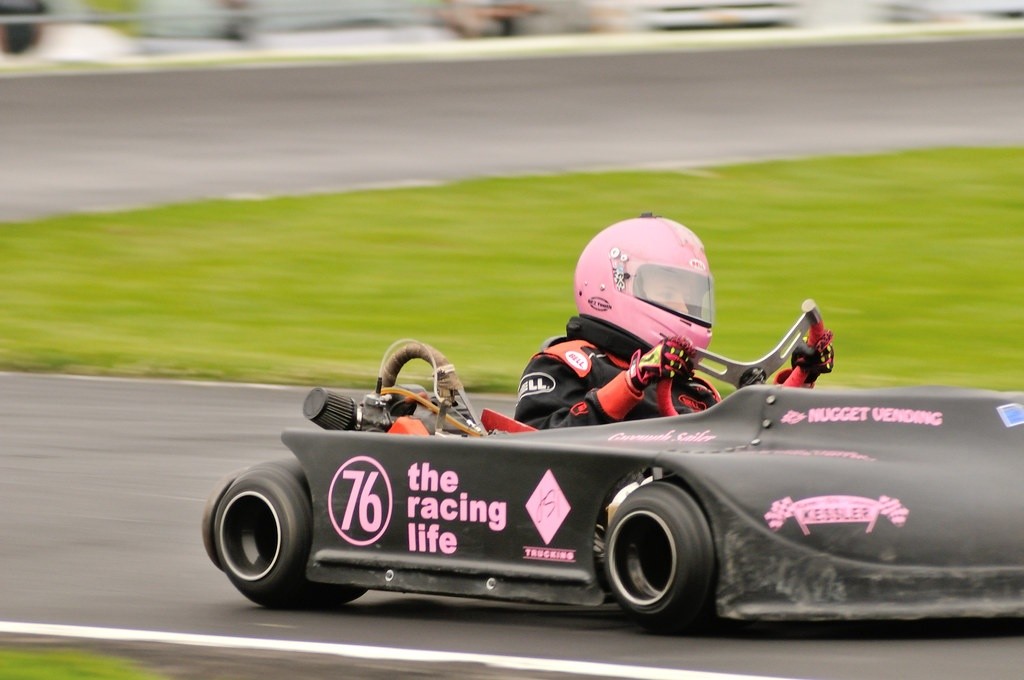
left=789, top=329, right=833, bottom=383
left=626, top=335, right=695, bottom=391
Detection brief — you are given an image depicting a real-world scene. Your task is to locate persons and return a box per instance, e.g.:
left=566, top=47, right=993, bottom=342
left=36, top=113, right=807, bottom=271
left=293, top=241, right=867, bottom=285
left=515, top=216, right=834, bottom=428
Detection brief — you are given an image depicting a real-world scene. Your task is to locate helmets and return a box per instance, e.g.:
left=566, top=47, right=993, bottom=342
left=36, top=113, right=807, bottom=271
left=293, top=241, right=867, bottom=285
left=574, top=212, right=711, bottom=368
